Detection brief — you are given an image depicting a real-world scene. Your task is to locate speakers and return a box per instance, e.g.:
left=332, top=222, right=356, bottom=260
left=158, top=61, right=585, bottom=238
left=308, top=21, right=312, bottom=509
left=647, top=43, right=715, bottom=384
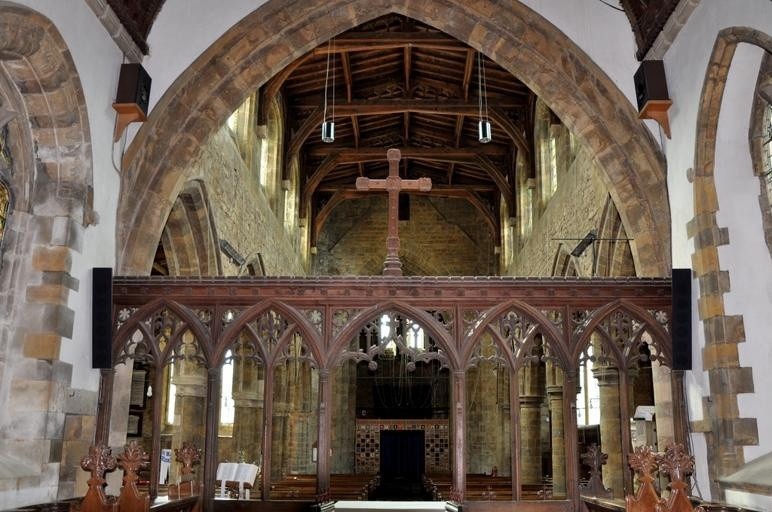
left=116, top=63, right=152, bottom=116
left=634, top=59, right=669, bottom=112
left=672, top=268, right=692, bottom=370
left=92, top=267, right=111, bottom=368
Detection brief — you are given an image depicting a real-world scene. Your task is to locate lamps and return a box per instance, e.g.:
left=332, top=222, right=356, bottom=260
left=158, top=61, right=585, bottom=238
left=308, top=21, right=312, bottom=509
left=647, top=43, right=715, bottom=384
left=320, top=37, right=336, bottom=146
left=476, top=49, right=492, bottom=146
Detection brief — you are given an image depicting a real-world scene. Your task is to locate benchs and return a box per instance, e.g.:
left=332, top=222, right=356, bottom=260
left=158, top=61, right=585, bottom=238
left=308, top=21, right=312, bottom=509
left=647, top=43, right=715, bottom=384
left=249, top=469, right=556, bottom=504
